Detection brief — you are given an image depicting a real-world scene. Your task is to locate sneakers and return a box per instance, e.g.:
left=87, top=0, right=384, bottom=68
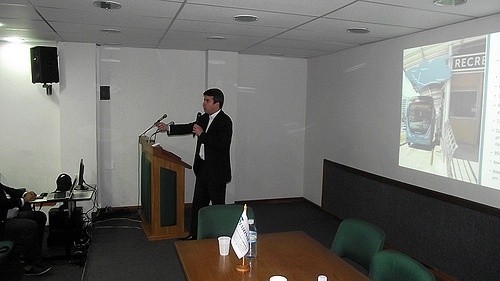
left=24, top=265, right=53, bottom=276
left=19, top=256, right=24, bottom=263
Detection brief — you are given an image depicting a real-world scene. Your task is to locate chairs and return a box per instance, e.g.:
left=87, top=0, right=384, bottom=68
left=330, top=217, right=437, bottom=281
left=194, top=204, right=256, bottom=240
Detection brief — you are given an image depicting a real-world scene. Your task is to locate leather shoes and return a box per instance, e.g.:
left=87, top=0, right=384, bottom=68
left=177, top=234, right=193, bottom=240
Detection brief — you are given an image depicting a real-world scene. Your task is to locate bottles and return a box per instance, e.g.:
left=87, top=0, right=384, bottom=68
left=245, top=219, right=258, bottom=258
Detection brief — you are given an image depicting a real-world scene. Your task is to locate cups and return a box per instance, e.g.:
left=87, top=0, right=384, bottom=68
left=217, top=236, right=230, bottom=256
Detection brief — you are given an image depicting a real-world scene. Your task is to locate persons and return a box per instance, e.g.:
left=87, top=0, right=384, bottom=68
left=156, top=87, right=233, bottom=241
left=1, top=182, right=53, bottom=275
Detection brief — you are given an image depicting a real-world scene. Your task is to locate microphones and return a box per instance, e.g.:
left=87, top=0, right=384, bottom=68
left=140, top=113, right=167, bottom=136
left=193, top=112, right=202, bottom=138
left=150, top=121, right=174, bottom=139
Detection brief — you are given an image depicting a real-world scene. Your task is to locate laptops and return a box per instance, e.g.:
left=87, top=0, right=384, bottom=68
left=46, top=175, right=78, bottom=201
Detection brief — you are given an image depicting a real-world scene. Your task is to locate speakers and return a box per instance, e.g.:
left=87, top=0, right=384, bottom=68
left=30, top=46, right=59, bottom=84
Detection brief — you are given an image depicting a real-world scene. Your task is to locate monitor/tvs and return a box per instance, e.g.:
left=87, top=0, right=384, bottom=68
left=78, top=159, right=84, bottom=185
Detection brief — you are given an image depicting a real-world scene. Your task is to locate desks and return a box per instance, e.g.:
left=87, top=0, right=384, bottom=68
left=30, top=188, right=96, bottom=258
left=174, top=227, right=375, bottom=281
